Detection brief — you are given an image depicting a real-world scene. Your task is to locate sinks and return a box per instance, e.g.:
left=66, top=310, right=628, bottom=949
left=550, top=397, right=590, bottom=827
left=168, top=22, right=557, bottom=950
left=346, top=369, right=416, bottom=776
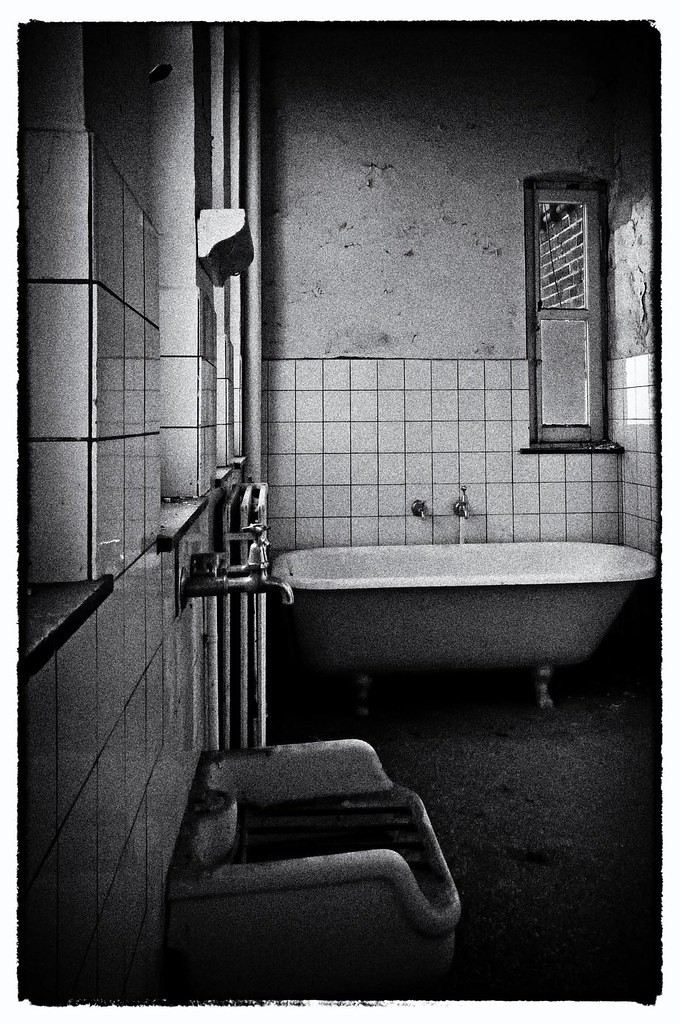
left=162, top=737, right=462, bottom=1000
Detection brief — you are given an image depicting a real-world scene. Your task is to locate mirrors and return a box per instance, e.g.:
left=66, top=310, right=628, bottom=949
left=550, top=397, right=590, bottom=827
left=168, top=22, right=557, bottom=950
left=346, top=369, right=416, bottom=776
left=523, top=173, right=608, bottom=446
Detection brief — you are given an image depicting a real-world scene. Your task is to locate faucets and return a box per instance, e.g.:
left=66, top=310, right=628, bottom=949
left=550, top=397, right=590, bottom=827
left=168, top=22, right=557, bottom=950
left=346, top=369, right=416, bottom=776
left=453, top=485, right=469, bottom=520
left=179, top=523, right=294, bottom=612
left=417, top=499, right=428, bottom=522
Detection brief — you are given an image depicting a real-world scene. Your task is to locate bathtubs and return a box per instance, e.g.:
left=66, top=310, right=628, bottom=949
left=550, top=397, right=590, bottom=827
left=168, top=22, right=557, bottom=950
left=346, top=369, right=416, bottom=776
left=272, top=541, right=656, bottom=710
